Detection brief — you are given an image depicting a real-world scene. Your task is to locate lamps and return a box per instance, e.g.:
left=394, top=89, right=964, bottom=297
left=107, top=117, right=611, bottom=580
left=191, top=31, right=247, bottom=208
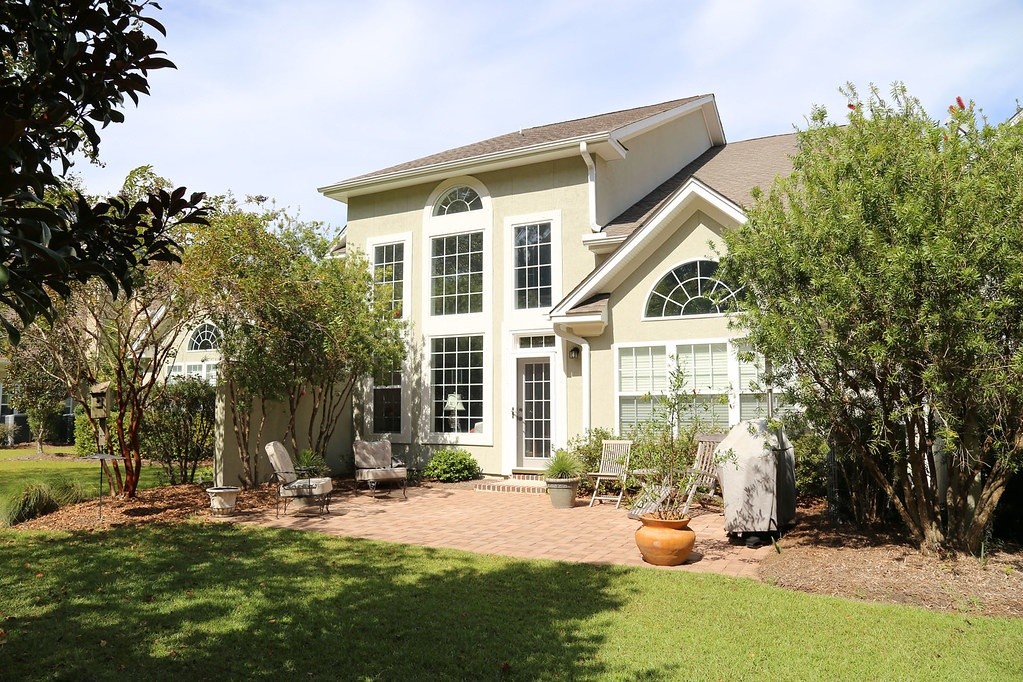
left=568, top=347, right=580, bottom=359
left=443, top=394, right=466, bottom=433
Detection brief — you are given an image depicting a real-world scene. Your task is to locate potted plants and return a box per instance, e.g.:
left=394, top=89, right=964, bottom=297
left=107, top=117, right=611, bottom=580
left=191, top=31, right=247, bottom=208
left=293, top=449, right=331, bottom=478
left=614, top=351, right=742, bottom=569
left=542, top=449, right=584, bottom=509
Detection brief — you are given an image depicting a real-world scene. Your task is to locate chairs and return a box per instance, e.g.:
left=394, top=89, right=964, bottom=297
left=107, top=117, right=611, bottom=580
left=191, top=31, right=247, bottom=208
left=352, top=440, right=408, bottom=500
left=264, top=441, right=333, bottom=518
left=586, top=439, right=633, bottom=509
left=627, top=433, right=726, bottom=519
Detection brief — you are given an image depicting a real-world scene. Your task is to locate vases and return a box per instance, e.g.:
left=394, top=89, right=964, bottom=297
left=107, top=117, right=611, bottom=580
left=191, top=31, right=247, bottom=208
left=205, top=486, right=241, bottom=518
